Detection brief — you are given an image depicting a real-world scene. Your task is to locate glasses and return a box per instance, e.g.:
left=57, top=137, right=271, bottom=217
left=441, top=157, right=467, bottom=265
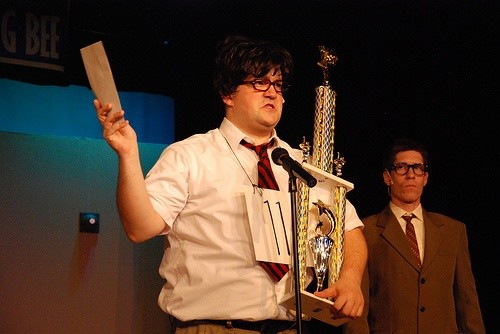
left=389, top=161, right=427, bottom=176
left=236, top=76, right=291, bottom=93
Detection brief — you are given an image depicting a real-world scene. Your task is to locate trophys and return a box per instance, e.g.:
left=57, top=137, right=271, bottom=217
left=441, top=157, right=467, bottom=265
left=277, top=46, right=351, bottom=327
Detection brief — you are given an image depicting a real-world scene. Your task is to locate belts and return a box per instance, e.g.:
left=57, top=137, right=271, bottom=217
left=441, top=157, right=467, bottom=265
left=173, top=318, right=297, bottom=331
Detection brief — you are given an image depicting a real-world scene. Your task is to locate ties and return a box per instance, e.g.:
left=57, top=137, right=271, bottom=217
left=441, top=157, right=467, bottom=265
left=239, top=137, right=290, bottom=284
left=400, top=213, right=422, bottom=270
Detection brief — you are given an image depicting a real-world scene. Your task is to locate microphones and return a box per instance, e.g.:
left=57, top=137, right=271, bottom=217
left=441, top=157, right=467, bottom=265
left=271, top=147, right=317, bottom=188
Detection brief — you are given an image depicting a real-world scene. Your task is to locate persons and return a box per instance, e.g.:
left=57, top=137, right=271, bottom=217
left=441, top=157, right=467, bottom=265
left=346, top=141, right=486, bottom=334
left=94, top=36, right=368, bottom=334
